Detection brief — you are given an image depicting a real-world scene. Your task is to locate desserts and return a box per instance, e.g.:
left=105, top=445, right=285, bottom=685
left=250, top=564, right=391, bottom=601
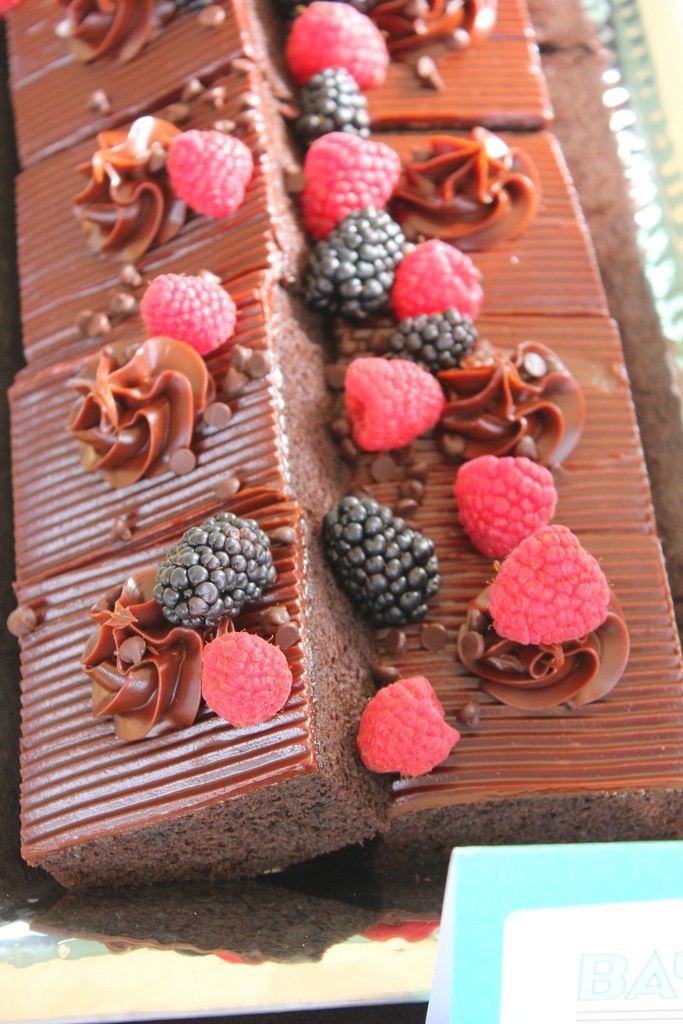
left=0, top=0, right=683, bottom=908
